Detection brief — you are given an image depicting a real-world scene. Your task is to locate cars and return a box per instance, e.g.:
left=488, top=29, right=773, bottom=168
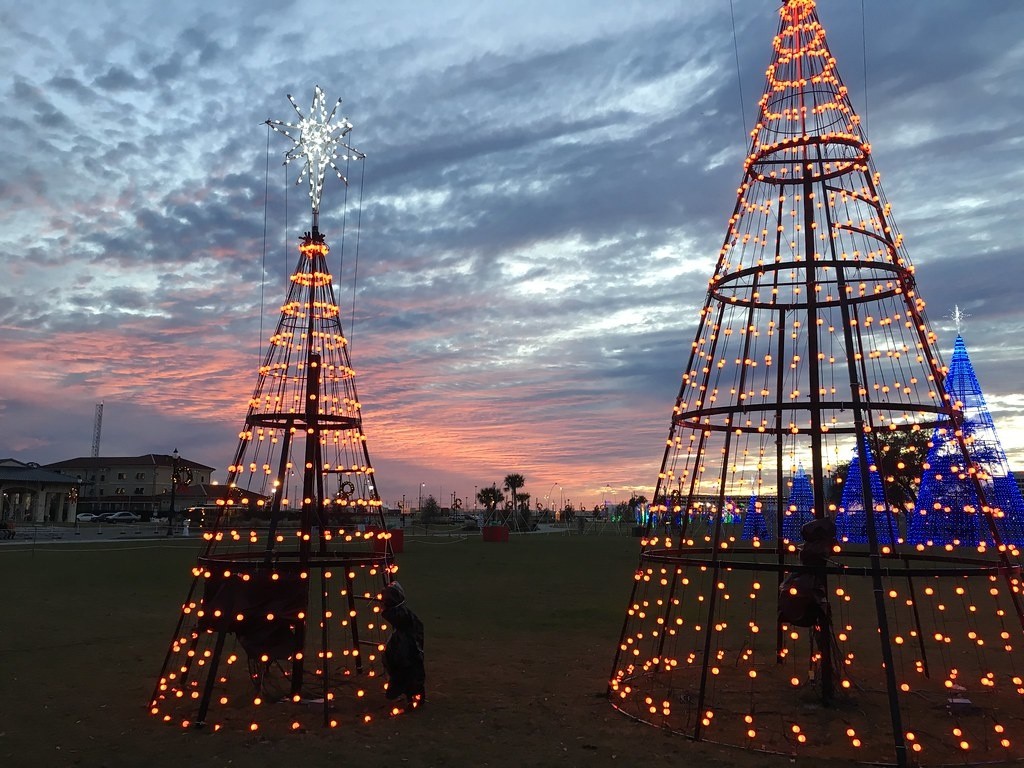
left=105, top=511, right=141, bottom=524
left=76, top=512, right=97, bottom=522
left=92, top=513, right=116, bottom=523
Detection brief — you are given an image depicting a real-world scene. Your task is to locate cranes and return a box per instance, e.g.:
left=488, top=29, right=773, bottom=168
left=91, top=401, right=103, bottom=458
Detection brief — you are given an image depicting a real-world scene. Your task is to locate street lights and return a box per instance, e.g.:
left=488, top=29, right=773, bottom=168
left=271, top=489, right=277, bottom=503
left=474, top=485, right=478, bottom=511
left=294, top=485, right=298, bottom=509
left=419, top=480, right=426, bottom=512
left=547, top=482, right=558, bottom=510
left=165, top=448, right=180, bottom=537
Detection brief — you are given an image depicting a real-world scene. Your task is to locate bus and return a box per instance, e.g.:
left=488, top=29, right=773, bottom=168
left=182, top=503, right=251, bottom=529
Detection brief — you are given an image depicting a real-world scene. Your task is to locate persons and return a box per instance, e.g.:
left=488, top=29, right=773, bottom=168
left=0, top=518, right=16, bottom=540
